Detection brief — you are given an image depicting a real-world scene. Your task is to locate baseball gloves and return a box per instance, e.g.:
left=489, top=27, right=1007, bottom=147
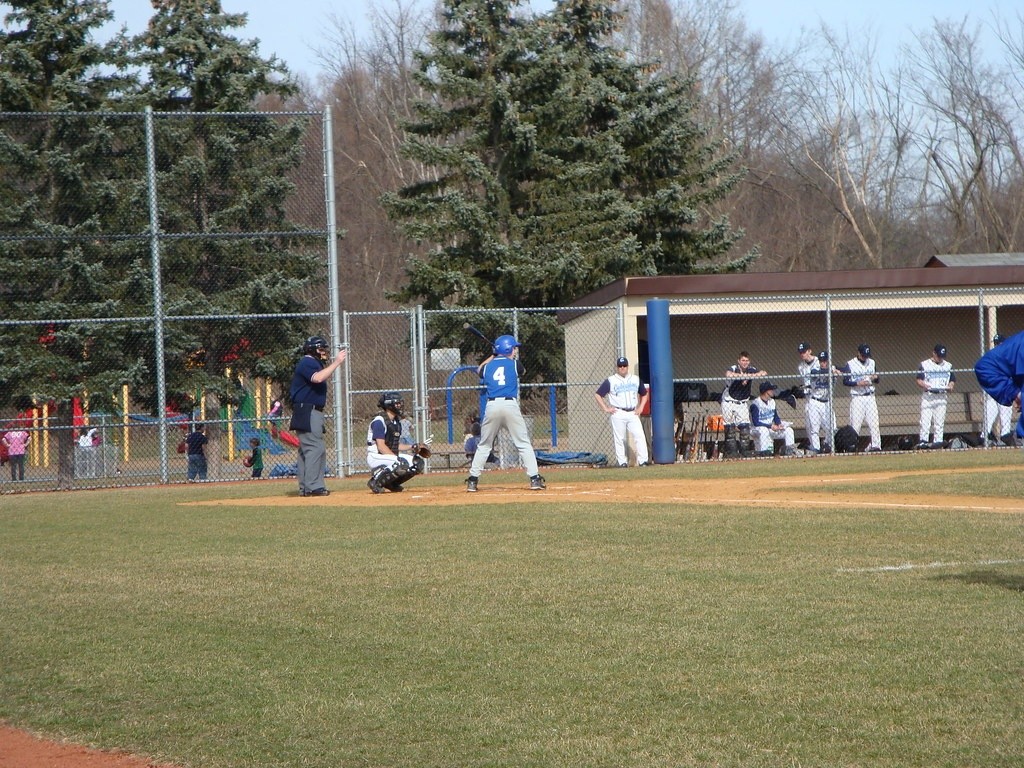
left=412, top=442, right=432, bottom=458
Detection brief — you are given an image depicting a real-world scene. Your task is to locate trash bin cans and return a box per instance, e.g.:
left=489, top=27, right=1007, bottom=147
left=219, top=387, right=254, bottom=431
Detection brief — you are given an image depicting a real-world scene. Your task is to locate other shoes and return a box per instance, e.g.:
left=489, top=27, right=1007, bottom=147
left=621, top=463, right=627, bottom=468
left=639, top=462, right=648, bottom=467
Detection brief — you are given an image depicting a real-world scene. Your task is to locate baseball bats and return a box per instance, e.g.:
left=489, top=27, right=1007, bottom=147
left=463, top=322, right=494, bottom=347
left=673, top=414, right=722, bottom=463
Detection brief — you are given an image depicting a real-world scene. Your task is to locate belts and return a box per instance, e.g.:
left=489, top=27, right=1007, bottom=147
left=925, top=390, right=946, bottom=394
left=726, top=400, right=746, bottom=404
left=616, top=407, right=635, bottom=412
left=811, top=396, right=828, bottom=402
left=489, top=397, right=513, bottom=400
left=853, top=392, right=873, bottom=396
left=292, top=404, right=322, bottom=412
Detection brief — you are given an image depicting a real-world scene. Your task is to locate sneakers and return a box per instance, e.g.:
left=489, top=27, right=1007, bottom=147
left=464, top=477, right=477, bottom=491
left=531, top=474, right=546, bottom=490
left=367, top=476, right=384, bottom=493
left=298, top=487, right=330, bottom=496
left=383, top=483, right=402, bottom=491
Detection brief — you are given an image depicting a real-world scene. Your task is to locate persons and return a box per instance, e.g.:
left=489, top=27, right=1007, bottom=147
left=467, top=335, right=546, bottom=492
left=366, top=392, right=432, bottom=494
left=74, top=426, right=97, bottom=447
left=596, top=357, right=649, bottom=467
left=844, top=343, right=882, bottom=451
left=266, top=393, right=282, bottom=424
left=797, top=342, right=841, bottom=453
left=185, top=424, right=208, bottom=480
left=2, top=424, right=32, bottom=480
left=915, top=344, right=955, bottom=449
left=399, top=413, right=415, bottom=454
left=464, top=422, right=499, bottom=464
left=749, top=381, right=800, bottom=457
left=975, top=330, right=1024, bottom=446
left=721, top=352, right=767, bottom=458
left=289, top=336, right=346, bottom=496
left=245, top=438, right=264, bottom=477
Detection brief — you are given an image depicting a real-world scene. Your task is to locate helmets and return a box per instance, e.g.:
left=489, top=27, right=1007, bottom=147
left=303, top=337, right=328, bottom=359
left=377, top=391, right=405, bottom=416
left=897, top=434, right=913, bottom=450
left=495, top=335, right=521, bottom=353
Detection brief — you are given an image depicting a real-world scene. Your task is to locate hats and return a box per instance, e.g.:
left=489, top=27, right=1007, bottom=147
left=617, top=357, right=628, bottom=366
left=796, top=343, right=810, bottom=353
left=991, top=334, right=1004, bottom=342
left=818, top=352, right=828, bottom=361
left=934, top=345, right=947, bottom=357
left=759, top=382, right=777, bottom=394
left=858, top=344, right=872, bottom=358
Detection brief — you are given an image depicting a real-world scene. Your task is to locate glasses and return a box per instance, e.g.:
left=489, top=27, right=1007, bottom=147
left=618, top=365, right=626, bottom=367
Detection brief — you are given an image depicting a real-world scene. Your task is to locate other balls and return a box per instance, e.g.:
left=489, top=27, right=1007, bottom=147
left=243, top=456, right=253, bottom=467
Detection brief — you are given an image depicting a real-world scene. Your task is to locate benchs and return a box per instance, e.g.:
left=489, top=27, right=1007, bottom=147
left=681, top=390, right=1021, bottom=453
left=430, top=448, right=548, bottom=468
